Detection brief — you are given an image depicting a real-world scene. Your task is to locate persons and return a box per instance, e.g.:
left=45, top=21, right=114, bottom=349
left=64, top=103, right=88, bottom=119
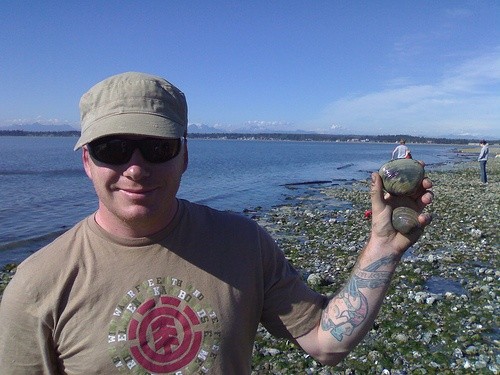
left=0, top=72, right=434, bottom=375
left=391, top=137, right=411, bottom=159
left=476, top=138, right=490, bottom=185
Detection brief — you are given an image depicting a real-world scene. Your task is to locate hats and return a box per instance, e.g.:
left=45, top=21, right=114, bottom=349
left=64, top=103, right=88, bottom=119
left=73, top=72, right=188, bottom=151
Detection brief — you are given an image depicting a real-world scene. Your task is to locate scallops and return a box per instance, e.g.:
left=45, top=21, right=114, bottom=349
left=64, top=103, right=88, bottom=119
left=391, top=206, right=423, bottom=234
left=379, top=158, right=424, bottom=197
left=0, top=146, right=500, bottom=375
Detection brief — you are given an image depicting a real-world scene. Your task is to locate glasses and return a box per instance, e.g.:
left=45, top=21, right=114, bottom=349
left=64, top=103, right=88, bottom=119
left=87, top=135, right=187, bottom=165
